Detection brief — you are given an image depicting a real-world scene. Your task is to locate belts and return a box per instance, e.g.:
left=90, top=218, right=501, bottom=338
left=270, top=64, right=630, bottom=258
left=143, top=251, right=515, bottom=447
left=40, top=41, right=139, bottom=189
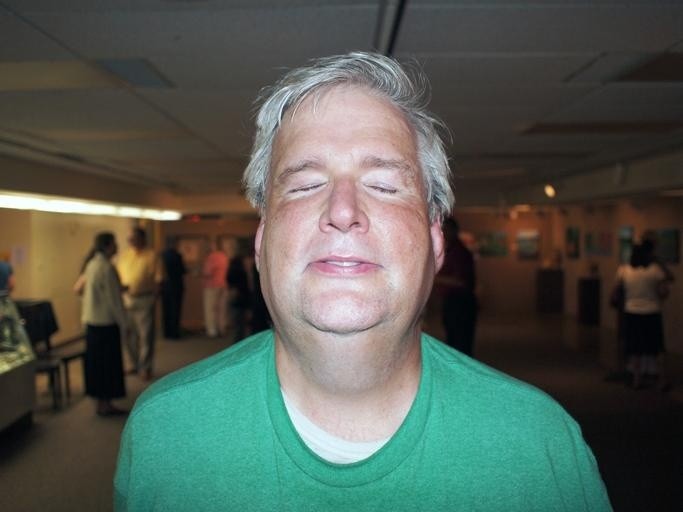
left=127, top=290, right=152, bottom=297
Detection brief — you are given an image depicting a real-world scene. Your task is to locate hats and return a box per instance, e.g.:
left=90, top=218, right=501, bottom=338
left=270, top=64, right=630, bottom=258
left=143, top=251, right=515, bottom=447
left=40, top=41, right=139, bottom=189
left=0, top=262, right=12, bottom=289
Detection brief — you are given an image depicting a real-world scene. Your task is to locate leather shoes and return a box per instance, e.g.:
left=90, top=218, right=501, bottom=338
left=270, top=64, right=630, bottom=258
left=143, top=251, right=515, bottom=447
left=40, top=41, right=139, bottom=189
left=97, top=405, right=123, bottom=418
left=126, top=366, right=151, bottom=380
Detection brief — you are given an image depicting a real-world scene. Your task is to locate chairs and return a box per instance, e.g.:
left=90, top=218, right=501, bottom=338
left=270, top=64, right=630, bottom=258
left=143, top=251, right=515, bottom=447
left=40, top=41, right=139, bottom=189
left=18, top=297, right=87, bottom=410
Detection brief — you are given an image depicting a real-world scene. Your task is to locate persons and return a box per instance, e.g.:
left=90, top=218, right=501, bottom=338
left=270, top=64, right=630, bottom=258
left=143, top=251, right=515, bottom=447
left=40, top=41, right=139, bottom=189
left=74, top=227, right=271, bottom=416
left=113, top=49, right=613, bottom=512
left=607, top=229, right=673, bottom=391
left=434, top=217, right=480, bottom=358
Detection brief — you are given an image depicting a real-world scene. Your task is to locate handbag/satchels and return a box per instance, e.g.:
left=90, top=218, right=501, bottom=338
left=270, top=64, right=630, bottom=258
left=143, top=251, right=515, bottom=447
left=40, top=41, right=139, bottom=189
left=608, top=284, right=623, bottom=311
left=73, top=277, right=85, bottom=296
